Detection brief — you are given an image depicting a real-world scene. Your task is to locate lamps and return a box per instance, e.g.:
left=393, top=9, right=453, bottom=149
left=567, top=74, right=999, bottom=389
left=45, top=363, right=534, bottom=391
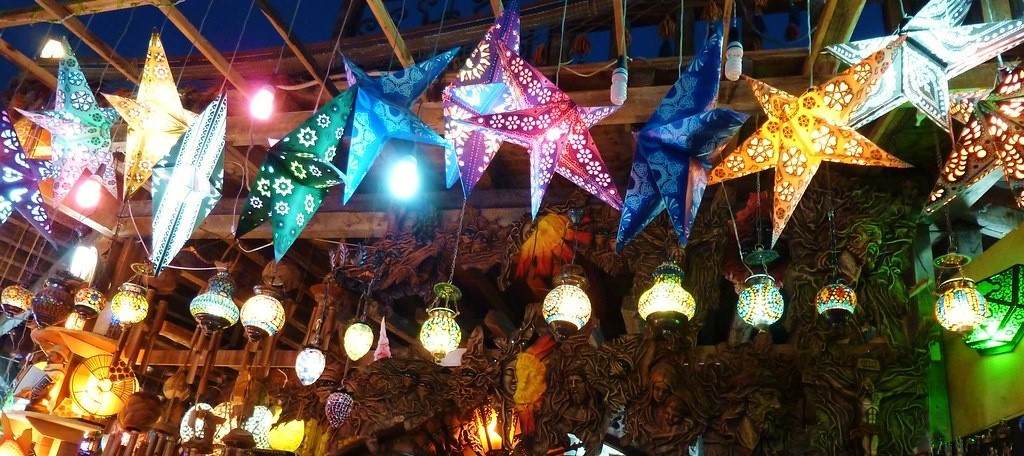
left=189, top=270, right=238, bottom=335
left=344, top=281, right=374, bottom=363
left=110, top=259, right=152, bottom=330
left=295, top=295, right=326, bottom=385
left=419, top=198, right=467, bottom=363
left=75, top=286, right=106, bottom=321
left=240, top=283, right=289, bottom=343
left=638, top=252, right=697, bottom=325
left=541, top=207, right=594, bottom=337
left=934, top=231, right=991, bottom=335
left=812, top=163, right=860, bottom=319
left=172, top=397, right=316, bottom=454
left=31, top=275, right=74, bottom=328
left=731, top=171, right=790, bottom=332
left=1, top=282, right=32, bottom=323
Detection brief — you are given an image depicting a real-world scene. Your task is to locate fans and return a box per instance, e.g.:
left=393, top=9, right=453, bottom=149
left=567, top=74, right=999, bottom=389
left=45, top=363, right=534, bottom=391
left=72, top=352, right=138, bottom=422
